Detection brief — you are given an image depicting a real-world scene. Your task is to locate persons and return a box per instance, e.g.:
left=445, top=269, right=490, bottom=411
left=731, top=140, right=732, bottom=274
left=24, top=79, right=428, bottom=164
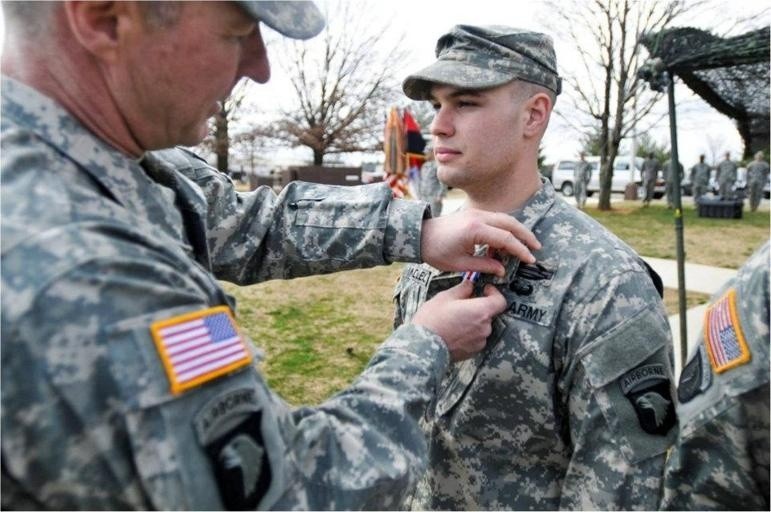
left=689, top=153, right=711, bottom=210
left=572, top=152, right=593, bottom=210
left=745, top=151, right=770, bottom=212
left=385, top=21, right=683, bottom=511
left=2, top=2, right=542, bottom=511
left=662, top=150, right=685, bottom=211
left=661, top=235, right=771, bottom=510
left=714, top=151, right=738, bottom=202
left=638, top=148, right=661, bottom=208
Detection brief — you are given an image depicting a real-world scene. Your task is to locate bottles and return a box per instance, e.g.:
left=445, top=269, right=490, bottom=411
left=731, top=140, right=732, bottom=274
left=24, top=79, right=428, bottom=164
left=402, top=23, right=563, bottom=102
left=644, top=149, right=765, bottom=161
left=235, top=1, right=327, bottom=41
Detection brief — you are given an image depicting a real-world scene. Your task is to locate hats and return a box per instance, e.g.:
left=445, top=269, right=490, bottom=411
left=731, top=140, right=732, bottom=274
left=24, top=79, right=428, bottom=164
left=679, top=165, right=771, bottom=200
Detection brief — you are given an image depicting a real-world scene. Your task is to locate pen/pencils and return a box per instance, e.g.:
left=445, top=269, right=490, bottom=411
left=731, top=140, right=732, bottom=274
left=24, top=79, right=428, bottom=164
left=549, top=154, right=665, bottom=200
